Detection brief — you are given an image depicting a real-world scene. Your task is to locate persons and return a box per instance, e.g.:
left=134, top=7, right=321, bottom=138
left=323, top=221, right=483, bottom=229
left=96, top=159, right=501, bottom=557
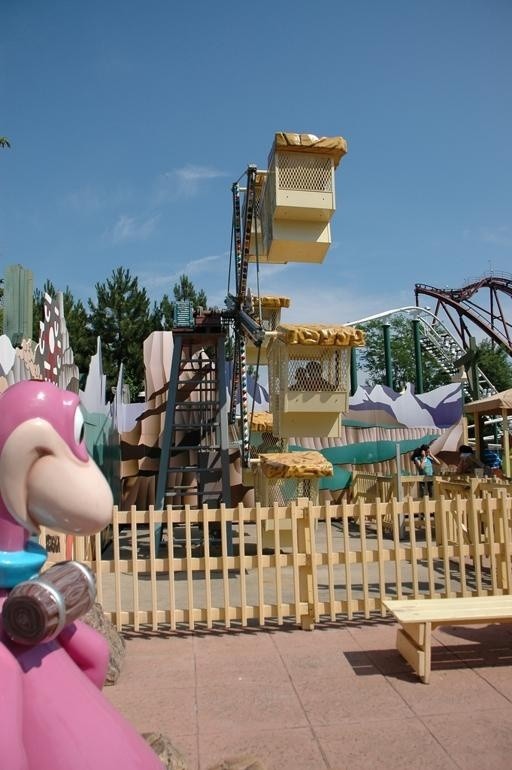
left=411, top=444, right=441, bottom=521
left=289, top=367, right=309, bottom=391
left=480, top=444, right=502, bottom=469
left=305, top=361, right=336, bottom=391
left=459, top=446, right=475, bottom=474
left=456, top=445, right=479, bottom=489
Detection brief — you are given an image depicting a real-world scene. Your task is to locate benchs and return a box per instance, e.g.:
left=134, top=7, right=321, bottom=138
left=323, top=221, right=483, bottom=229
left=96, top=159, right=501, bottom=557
left=383, top=594, right=512, bottom=684
